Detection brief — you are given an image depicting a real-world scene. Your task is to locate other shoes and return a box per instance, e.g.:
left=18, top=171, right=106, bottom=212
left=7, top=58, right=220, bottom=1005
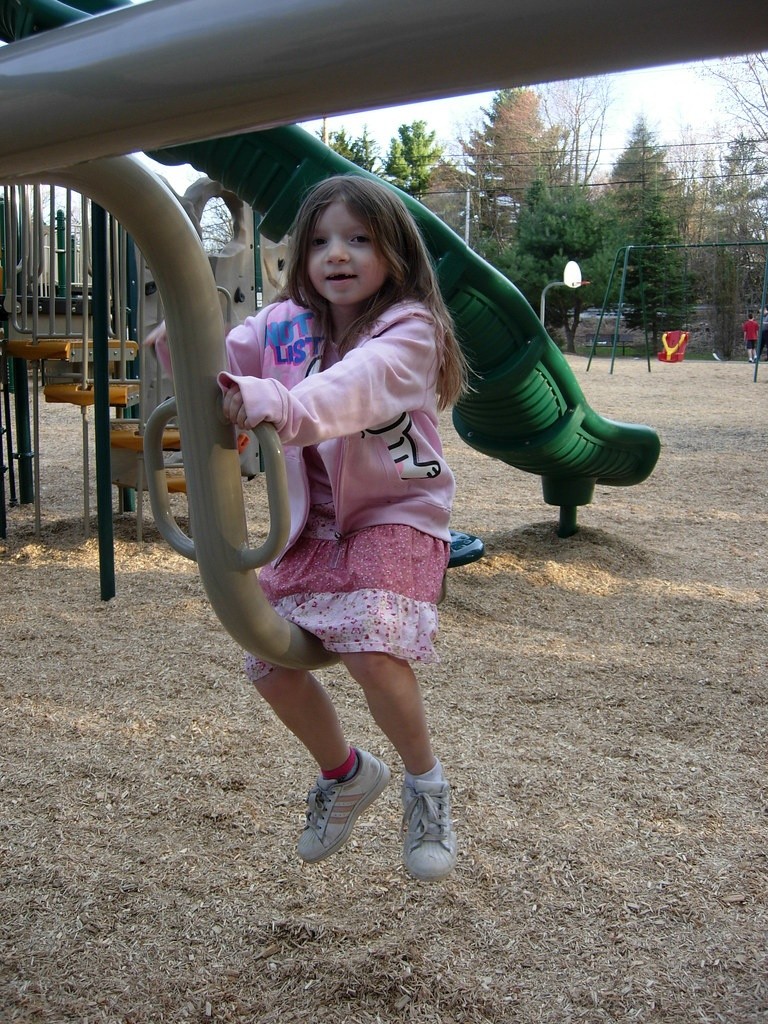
left=748, top=358, right=754, bottom=363
left=754, top=356, right=757, bottom=363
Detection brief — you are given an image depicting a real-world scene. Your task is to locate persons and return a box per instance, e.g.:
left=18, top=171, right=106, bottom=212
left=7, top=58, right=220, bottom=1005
left=142, top=177, right=485, bottom=881
left=744, top=304, right=768, bottom=363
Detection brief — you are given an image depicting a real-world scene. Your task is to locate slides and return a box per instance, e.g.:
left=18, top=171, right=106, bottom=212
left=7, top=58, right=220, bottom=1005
left=1, top=0, right=661, bottom=488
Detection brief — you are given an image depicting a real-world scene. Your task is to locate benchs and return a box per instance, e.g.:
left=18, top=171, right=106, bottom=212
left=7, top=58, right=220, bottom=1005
left=586, top=333, right=635, bottom=357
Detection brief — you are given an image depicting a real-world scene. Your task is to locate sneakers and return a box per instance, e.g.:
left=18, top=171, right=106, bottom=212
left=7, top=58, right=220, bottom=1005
left=401, top=777, right=458, bottom=881
left=297, top=746, right=391, bottom=863
